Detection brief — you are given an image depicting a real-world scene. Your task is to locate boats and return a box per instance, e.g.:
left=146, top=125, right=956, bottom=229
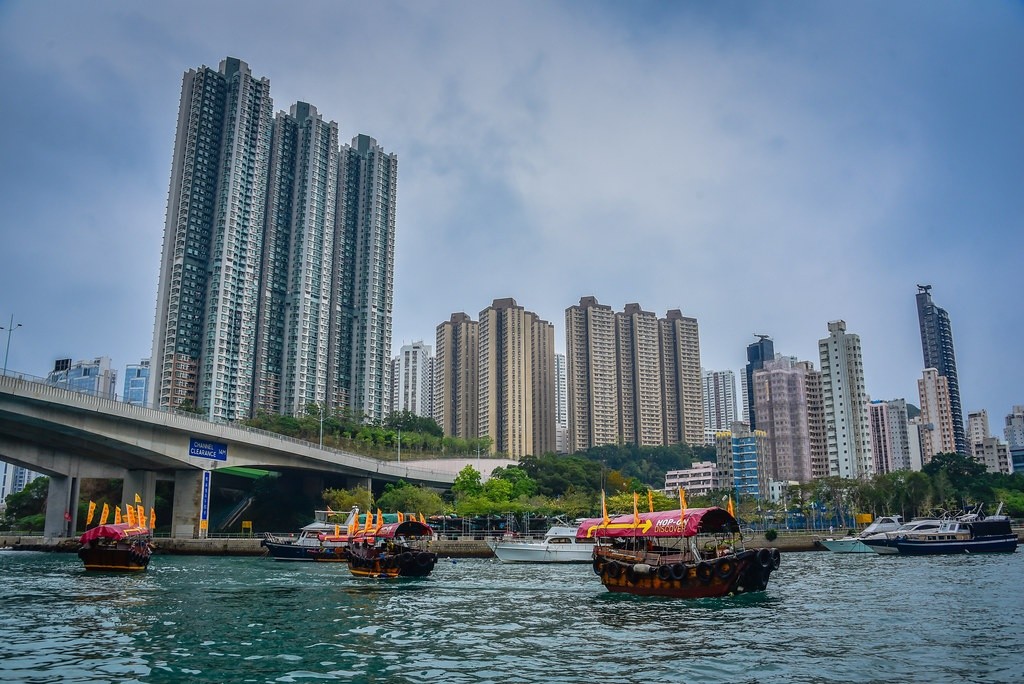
left=818, top=501, right=903, bottom=554
left=858, top=514, right=943, bottom=556
left=259, top=520, right=374, bottom=562
left=483, top=525, right=631, bottom=565
left=886, top=500, right=1018, bottom=556
left=343, top=521, right=439, bottom=578
left=575, top=506, right=781, bottom=598
left=77, top=522, right=153, bottom=571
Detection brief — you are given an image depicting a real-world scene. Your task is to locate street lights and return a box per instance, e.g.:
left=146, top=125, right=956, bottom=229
left=473, top=443, right=486, bottom=470
left=393, top=428, right=407, bottom=463
left=312, top=409, right=335, bottom=451
left=0, top=313, right=23, bottom=377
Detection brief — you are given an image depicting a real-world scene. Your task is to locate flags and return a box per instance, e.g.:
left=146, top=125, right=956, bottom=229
left=87, top=494, right=156, bottom=529
left=335, top=508, right=426, bottom=537
left=64, top=507, right=71, bottom=522
left=602, top=487, right=734, bottom=530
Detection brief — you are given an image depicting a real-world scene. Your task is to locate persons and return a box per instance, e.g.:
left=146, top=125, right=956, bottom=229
left=95, top=537, right=156, bottom=554
left=622, top=537, right=654, bottom=551
left=829, top=525, right=834, bottom=535
left=354, top=537, right=399, bottom=556
